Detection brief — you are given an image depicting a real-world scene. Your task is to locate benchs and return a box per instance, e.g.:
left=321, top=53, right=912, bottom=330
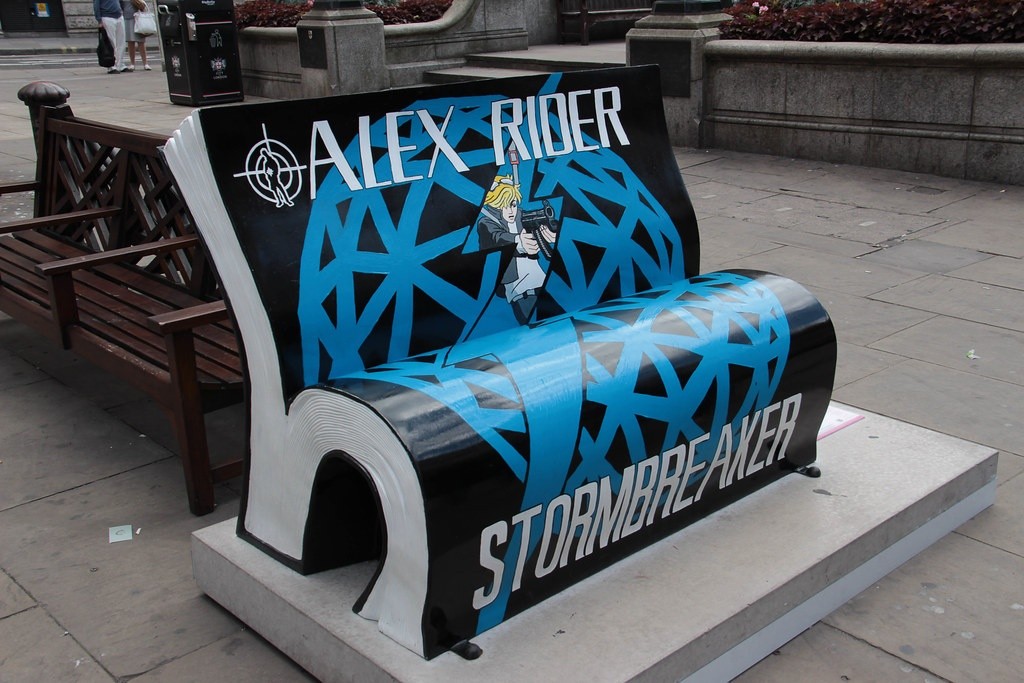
left=1, top=103, right=249, bottom=517
left=164, top=67, right=841, bottom=662
left=554, top=0, right=653, bottom=46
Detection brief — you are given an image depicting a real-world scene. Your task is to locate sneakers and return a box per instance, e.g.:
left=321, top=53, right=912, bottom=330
left=130, top=64, right=135, bottom=70
left=121, top=68, right=133, bottom=71
left=108, top=68, right=121, bottom=73
left=145, top=64, right=152, bottom=69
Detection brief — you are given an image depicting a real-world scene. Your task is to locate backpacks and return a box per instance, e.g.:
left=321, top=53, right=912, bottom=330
left=96, top=27, right=115, bottom=67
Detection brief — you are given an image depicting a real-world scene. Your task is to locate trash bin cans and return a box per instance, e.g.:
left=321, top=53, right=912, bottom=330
left=153, top=0, right=246, bottom=106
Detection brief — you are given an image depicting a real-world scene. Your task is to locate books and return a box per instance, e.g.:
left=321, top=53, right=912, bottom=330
left=162, top=62, right=839, bottom=662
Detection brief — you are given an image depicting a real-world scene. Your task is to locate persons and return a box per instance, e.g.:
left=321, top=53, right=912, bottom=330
left=92, top=0, right=133, bottom=74
left=120, top=0, right=152, bottom=72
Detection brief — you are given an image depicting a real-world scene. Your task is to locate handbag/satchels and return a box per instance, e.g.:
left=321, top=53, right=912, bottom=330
left=133, top=4, right=157, bottom=36
